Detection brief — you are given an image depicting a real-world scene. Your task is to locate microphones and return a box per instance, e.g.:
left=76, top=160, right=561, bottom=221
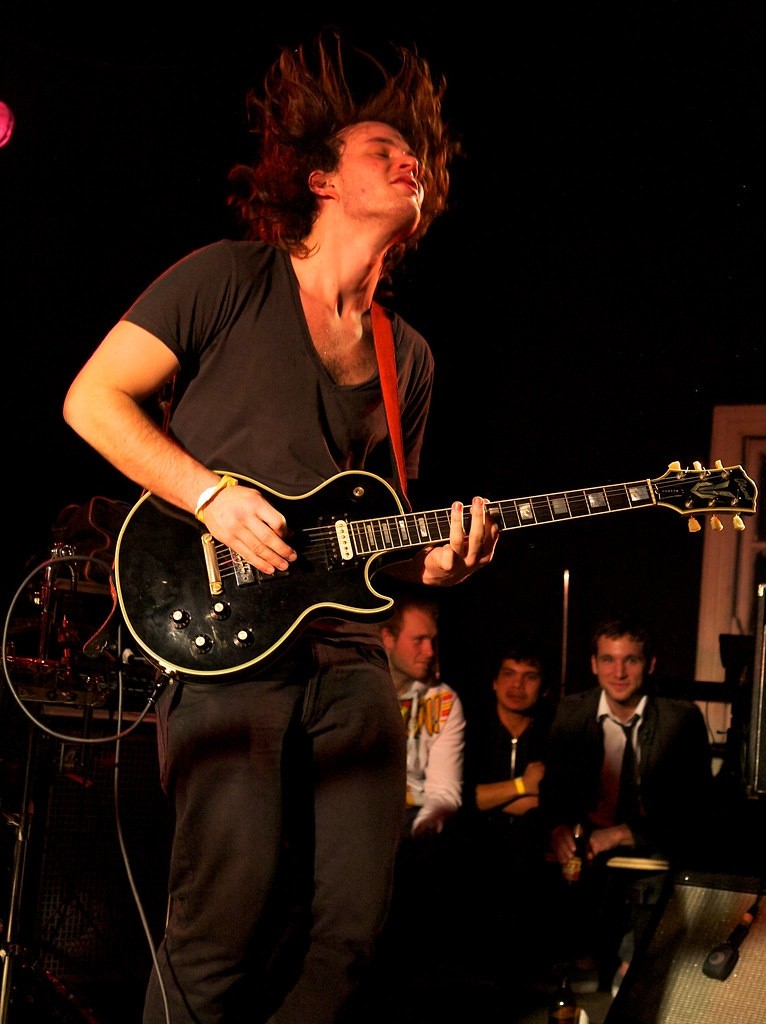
left=701, top=904, right=760, bottom=981
left=0, top=101, right=15, bottom=146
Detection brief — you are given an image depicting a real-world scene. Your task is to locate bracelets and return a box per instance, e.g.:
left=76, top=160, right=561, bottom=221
left=195, top=475, right=238, bottom=524
left=515, top=778, right=526, bottom=796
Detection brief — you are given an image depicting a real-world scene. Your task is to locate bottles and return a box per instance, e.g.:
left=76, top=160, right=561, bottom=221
left=548, top=978, right=578, bottom=1024
left=561, top=824, right=587, bottom=883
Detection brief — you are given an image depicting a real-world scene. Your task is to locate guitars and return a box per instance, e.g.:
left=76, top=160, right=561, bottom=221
left=119, top=457, right=761, bottom=680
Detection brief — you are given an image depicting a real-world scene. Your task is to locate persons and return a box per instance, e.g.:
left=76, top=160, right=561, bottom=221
left=62, top=36, right=459, bottom=1024
left=381, top=593, right=712, bottom=1024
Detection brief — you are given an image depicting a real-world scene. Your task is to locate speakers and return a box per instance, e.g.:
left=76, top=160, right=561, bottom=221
left=0, top=702, right=180, bottom=1024
left=605, top=870, right=765, bottom=1024
